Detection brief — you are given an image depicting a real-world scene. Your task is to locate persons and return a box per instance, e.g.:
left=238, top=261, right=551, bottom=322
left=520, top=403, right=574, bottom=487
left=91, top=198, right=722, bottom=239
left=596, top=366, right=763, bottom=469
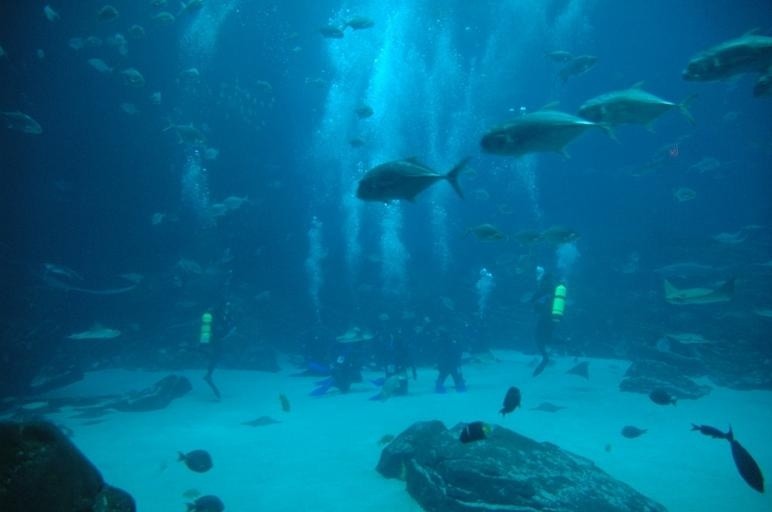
left=188, top=301, right=239, bottom=397
left=527, top=266, right=559, bottom=375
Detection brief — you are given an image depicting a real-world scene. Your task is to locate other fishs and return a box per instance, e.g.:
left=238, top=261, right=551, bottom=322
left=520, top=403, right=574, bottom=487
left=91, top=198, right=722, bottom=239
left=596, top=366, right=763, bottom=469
left=0, top=0, right=772, bottom=511
